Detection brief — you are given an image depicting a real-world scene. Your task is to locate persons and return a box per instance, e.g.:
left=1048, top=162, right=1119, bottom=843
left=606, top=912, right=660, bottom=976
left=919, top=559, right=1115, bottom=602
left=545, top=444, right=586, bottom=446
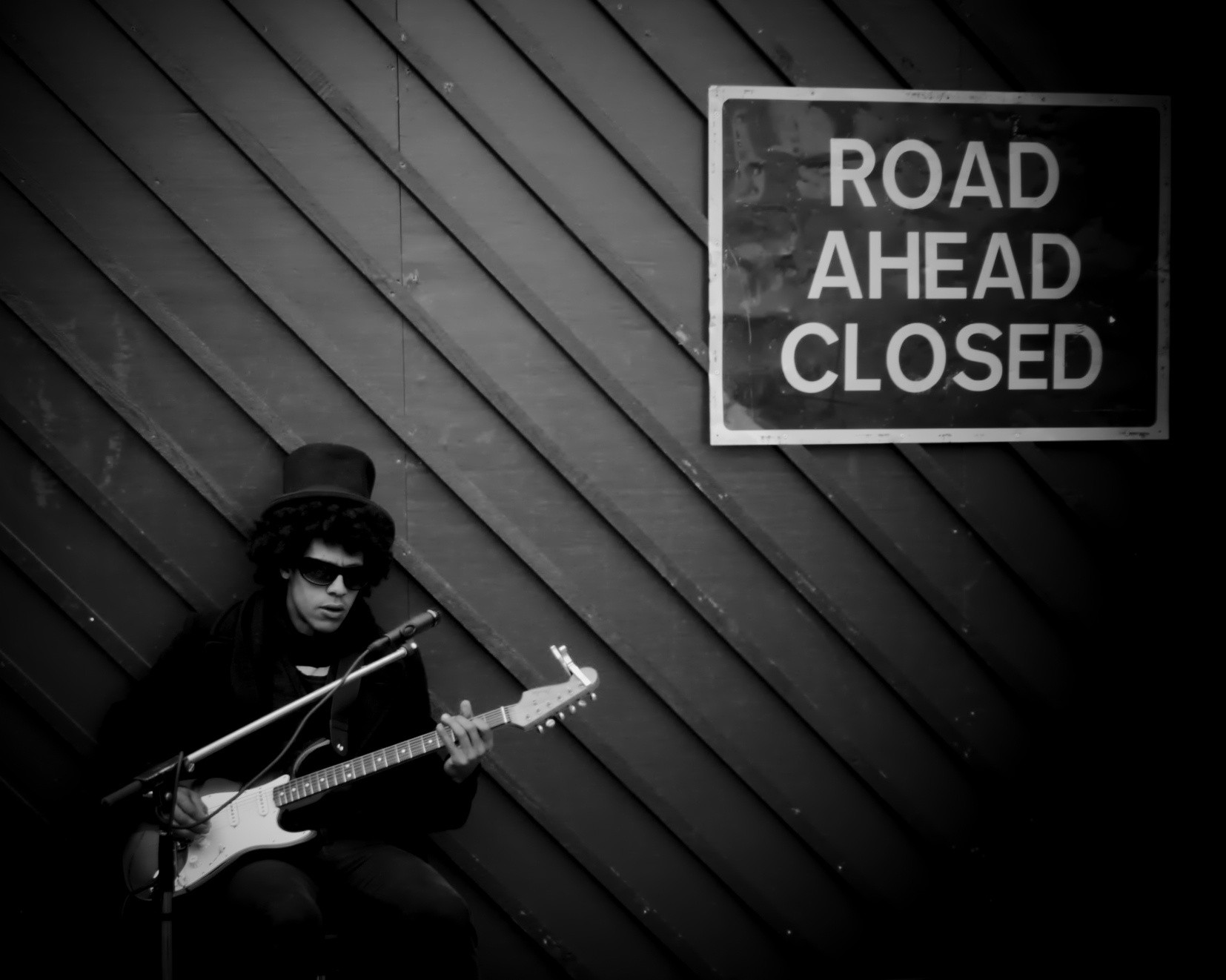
left=60, top=444, right=494, bottom=980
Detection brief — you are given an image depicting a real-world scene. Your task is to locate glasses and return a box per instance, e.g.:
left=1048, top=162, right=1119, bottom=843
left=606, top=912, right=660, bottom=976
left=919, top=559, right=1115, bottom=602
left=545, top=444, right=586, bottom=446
left=292, top=553, right=374, bottom=592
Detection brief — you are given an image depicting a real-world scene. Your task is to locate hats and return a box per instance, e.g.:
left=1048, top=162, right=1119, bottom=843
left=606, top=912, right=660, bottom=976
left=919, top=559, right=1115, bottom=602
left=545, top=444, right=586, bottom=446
left=261, top=443, right=396, bottom=546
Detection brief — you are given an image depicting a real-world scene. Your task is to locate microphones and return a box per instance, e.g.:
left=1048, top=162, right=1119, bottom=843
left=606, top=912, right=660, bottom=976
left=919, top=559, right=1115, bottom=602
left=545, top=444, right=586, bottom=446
left=367, top=606, right=443, bottom=654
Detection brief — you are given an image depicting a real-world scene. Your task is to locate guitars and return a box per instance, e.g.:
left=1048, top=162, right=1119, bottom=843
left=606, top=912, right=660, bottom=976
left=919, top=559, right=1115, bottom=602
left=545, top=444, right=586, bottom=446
left=120, top=644, right=602, bottom=906
